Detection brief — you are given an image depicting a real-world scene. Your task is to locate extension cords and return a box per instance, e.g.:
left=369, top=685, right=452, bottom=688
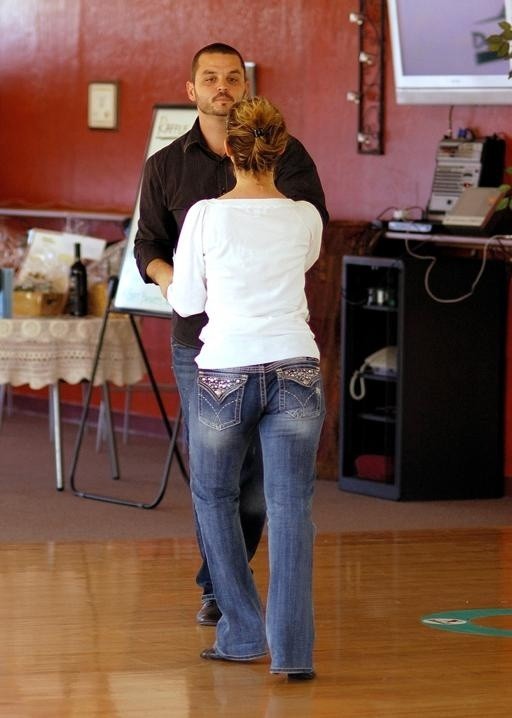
left=388, top=219, right=437, bottom=234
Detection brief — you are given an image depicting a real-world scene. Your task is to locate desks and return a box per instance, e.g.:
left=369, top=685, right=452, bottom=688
left=0, top=316, right=145, bottom=489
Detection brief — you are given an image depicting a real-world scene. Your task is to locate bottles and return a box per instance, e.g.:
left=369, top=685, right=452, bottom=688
left=368, top=288, right=386, bottom=305
left=67, top=243, right=88, bottom=318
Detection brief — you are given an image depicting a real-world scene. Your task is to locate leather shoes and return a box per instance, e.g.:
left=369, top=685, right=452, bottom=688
left=197, top=602, right=222, bottom=629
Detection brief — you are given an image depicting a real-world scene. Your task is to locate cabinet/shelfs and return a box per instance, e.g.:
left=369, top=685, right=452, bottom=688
left=339, top=253, right=509, bottom=501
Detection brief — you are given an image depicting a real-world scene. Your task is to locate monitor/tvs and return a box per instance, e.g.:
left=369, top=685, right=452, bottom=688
left=387, top=0, right=512, bottom=105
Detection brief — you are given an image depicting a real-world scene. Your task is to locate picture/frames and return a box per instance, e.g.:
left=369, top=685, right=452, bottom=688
left=386, top=0, right=512, bottom=89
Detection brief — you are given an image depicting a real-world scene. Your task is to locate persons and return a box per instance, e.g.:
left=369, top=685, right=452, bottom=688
left=168, top=97, right=325, bottom=680
left=133, top=43, right=330, bottom=626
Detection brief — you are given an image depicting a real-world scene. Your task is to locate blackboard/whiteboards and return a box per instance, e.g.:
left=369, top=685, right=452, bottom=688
left=111, top=103, right=199, bottom=319
